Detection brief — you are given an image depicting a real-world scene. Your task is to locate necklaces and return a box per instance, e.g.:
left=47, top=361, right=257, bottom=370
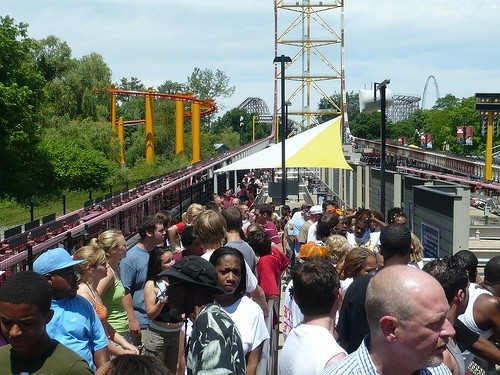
left=84, top=281, right=97, bottom=302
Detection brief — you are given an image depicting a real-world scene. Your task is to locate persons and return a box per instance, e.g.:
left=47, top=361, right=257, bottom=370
left=0, top=210, right=290, bottom=375
left=167, top=169, right=272, bottom=251
left=427, top=140, right=432, bottom=148
left=466, top=136, right=472, bottom=145
left=279, top=201, right=500, bottom=375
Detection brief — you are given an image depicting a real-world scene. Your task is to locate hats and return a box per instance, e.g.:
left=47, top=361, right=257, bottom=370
left=33, top=248, right=85, bottom=276
left=309, top=205, right=323, bottom=214
left=157, top=255, right=226, bottom=295
left=297, top=241, right=329, bottom=263
left=282, top=205, right=290, bottom=211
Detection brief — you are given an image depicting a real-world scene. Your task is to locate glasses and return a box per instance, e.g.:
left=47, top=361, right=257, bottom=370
left=164, top=280, right=186, bottom=290
